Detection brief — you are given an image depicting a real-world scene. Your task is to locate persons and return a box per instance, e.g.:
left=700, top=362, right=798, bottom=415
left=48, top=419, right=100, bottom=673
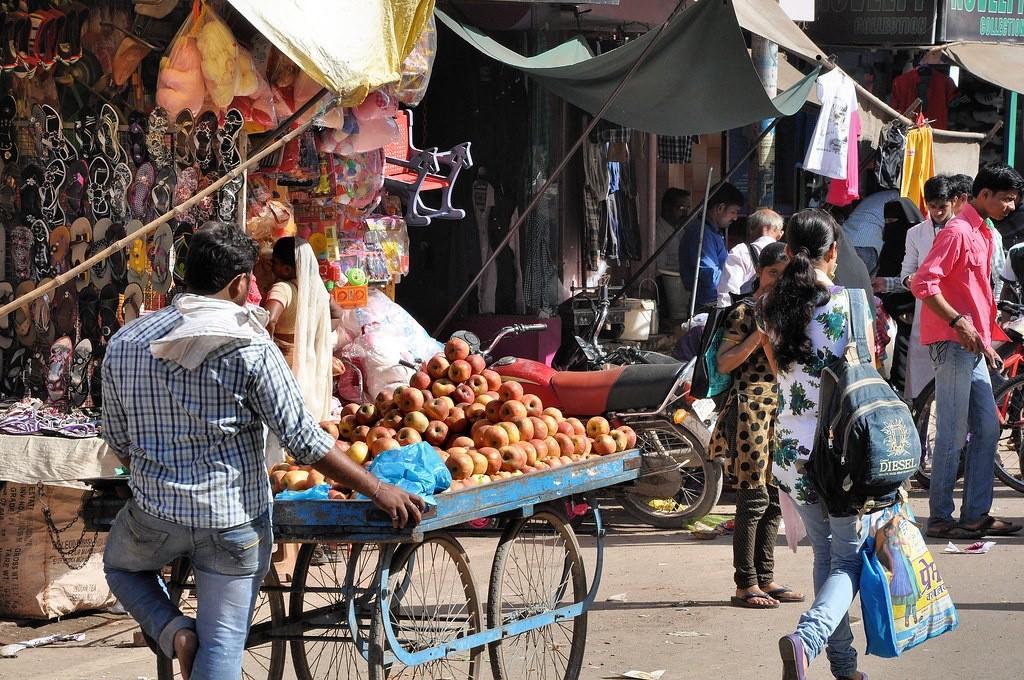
left=910, top=160, right=1024, bottom=539
left=678, top=181, right=745, bottom=322
left=716, top=208, right=785, bottom=408
left=841, top=189, right=901, bottom=277
left=706, top=242, right=791, bottom=609
left=101, top=221, right=425, bottom=680
left=264, top=236, right=343, bottom=471
left=754, top=208, right=877, bottom=680
left=871, top=173, right=1024, bottom=476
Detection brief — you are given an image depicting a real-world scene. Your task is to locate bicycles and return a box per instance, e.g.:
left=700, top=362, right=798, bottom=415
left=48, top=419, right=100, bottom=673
left=910, top=281, right=1024, bottom=493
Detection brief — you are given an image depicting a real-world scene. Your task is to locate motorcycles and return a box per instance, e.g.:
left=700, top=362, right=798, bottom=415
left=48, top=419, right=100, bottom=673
left=402, top=287, right=725, bottom=530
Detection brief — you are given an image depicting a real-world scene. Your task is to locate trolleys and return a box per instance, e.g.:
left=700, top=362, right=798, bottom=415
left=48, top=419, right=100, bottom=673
left=76, top=415, right=645, bottom=680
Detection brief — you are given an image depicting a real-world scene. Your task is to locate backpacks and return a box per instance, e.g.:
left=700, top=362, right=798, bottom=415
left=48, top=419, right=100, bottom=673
left=805, top=289, right=921, bottom=519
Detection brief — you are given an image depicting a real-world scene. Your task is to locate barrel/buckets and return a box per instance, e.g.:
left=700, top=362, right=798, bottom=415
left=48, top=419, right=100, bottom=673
left=616, top=298, right=654, bottom=341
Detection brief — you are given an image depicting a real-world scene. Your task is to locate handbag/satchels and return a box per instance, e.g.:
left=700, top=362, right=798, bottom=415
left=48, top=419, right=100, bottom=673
left=689, top=289, right=755, bottom=399
left=859, top=495, right=959, bottom=657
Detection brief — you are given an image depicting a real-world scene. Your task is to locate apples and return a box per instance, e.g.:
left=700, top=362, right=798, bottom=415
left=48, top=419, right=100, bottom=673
left=266, top=337, right=637, bottom=498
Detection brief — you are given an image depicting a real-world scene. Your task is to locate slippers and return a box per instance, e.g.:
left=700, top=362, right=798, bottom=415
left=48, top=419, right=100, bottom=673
left=926, top=522, right=986, bottom=540
left=0, top=0, right=245, bottom=438
left=967, top=515, right=1023, bottom=535
left=767, top=585, right=806, bottom=602
left=730, top=592, right=780, bottom=608
left=779, top=634, right=806, bottom=680
left=859, top=672, right=868, bottom=680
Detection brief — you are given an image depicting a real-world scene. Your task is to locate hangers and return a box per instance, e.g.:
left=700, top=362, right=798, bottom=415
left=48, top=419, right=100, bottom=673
left=915, top=63, right=932, bottom=76
left=892, top=110, right=903, bottom=126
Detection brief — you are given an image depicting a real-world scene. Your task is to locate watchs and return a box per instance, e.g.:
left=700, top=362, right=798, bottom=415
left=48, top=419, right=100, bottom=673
left=908, top=275, right=913, bottom=287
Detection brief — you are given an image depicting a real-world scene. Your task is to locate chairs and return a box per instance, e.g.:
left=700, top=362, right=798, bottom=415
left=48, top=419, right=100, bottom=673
left=386, top=108, right=473, bottom=226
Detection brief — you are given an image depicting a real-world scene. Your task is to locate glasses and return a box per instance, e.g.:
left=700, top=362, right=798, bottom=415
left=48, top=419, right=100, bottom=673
left=672, top=205, right=692, bottom=212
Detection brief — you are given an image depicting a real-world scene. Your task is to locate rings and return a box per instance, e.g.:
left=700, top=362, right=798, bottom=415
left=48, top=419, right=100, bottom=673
left=408, top=502, right=415, bottom=507
left=392, top=515, right=398, bottom=521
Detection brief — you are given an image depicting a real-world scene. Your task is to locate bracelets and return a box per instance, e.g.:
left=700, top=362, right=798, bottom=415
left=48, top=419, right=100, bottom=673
left=368, top=480, right=383, bottom=499
left=948, top=314, right=963, bottom=328
